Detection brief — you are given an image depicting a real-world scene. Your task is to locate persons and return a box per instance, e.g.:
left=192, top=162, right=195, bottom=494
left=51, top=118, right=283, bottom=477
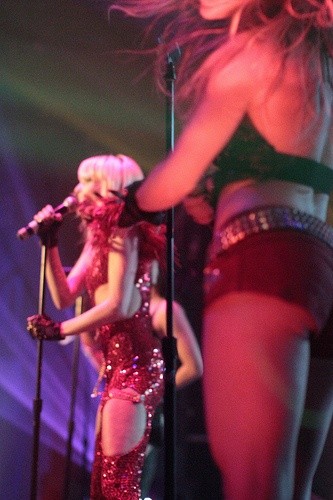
left=23, top=152, right=207, bottom=500
left=120, top=0, right=333, bottom=499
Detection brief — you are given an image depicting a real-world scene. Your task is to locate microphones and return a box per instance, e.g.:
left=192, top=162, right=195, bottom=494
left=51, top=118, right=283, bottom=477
left=17, top=196, right=79, bottom=241
